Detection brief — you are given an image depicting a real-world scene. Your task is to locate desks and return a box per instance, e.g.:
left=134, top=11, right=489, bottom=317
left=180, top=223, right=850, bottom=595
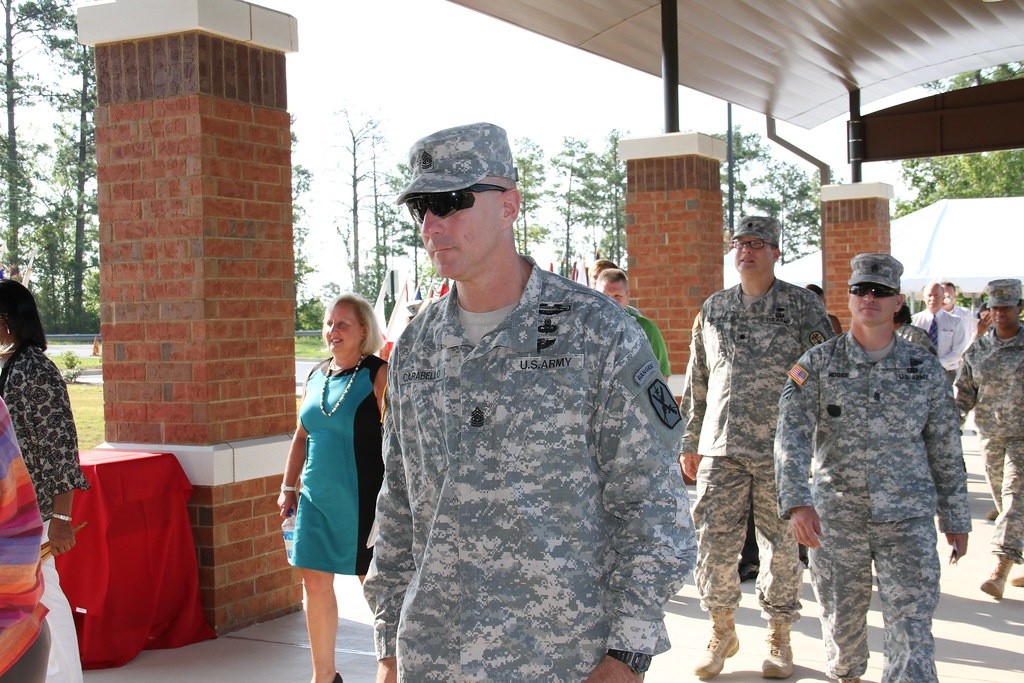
left=54, top=452, right=218, bottom=670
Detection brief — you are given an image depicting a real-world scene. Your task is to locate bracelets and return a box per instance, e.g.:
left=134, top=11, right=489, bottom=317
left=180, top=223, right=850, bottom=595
left=51, top=513, right=71, bottom=522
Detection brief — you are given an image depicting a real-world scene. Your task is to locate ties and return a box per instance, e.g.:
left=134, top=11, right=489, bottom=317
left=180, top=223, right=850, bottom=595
left=928, top=314, right=938, bottom=347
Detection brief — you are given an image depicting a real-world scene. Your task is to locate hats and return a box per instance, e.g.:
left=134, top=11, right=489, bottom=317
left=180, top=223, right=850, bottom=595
left=730, top=216, right=781, bottom=246
left=986, top=279, right=1022, bottom=308
left=848, top=253, right=904, bottom=293
left=396, top=122, right=519, bottom=207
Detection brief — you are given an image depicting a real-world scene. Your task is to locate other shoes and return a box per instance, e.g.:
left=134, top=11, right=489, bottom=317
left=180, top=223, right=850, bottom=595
left=986, top=509, right=998, bottom=519
left=333, top=672, right=343, bottom=683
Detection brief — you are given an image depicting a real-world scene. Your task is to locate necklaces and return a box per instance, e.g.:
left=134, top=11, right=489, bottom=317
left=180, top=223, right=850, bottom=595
left=320, top=354, right=365, bottom=416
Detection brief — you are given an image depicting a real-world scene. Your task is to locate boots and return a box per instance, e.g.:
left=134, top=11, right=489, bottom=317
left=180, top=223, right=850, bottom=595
left=762, top=621, right=795, bottom=679
left=1011, top=576, right=1024, bottom=586
left=693, top=609, right=739, bottom=679
left=980, top=556, right=1015, bottom=600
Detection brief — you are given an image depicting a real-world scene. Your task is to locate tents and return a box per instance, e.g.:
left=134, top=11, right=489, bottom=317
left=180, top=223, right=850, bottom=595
left=723, top=196, right=1024, bottom=289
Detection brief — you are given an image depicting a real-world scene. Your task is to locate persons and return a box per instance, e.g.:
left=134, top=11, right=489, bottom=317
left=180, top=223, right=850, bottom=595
left=587, top=259, right=670, bottom=387
left=277, top=294, right=393, bottom=683
left=892, top=282, right=993, bottom=392
left=803, top=283, right=841, bottom=339
left=773, top=252, right=972, bottom=683
left=676, top=214, right=837, bottom=679
left=0, top=399, right=53, bottom=683
left=0, top=278, right=90, bottom=683
left=953, top=280, right=1024, bottom=599
left=362, top=121, right=698, bottom=683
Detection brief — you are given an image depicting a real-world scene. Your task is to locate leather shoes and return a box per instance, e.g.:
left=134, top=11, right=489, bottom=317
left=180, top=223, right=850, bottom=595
left=738, top=561, right=760, bottom=582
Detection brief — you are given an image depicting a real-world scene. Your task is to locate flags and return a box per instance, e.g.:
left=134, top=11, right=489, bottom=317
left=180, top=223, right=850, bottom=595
left=370, top=273, right=449, bottom=364
left=547, top=251, right=590, bottom=286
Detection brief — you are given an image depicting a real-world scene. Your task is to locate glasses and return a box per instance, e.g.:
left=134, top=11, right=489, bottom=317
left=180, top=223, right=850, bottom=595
left=406, top=183, right=509, bottom=224
left=736, top=240, right=775, bottom=249
left=849, top=282, right=900, bottom=297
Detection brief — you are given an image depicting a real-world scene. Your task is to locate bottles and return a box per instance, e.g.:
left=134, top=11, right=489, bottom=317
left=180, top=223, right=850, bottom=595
left=282, top=507, right=297, bottom=566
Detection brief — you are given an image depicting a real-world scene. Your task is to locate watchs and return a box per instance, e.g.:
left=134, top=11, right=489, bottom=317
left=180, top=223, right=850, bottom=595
left=281, top=484, right=296, bottom=491
left=605, top=649, right=652, bottom=674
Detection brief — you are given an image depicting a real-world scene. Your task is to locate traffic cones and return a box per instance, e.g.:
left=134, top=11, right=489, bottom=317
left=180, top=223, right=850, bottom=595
left=89, top=336, right=102, bottom=356
